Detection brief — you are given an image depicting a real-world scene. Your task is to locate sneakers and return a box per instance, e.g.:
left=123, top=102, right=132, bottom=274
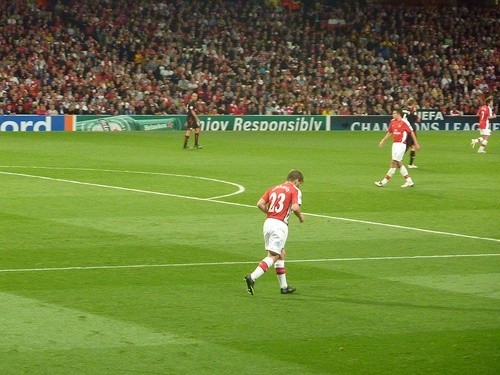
left=401, top=182, right=414, bottom=188
left=408, top=164, right=418, bottom=169
left=471, top=139, right=475, bottom=148
left=374, top=181, right=386, bottom=187
left=476, top=149, right=487, bottom=154
left=245, top=274, right=255, bottom=295
left=281, top=285, right=296, bottom=294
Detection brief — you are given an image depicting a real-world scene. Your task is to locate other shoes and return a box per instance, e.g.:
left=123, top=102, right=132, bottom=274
left=194, top=146, right=202, bottom=149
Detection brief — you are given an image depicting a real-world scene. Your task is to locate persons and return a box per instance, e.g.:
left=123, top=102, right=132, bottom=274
left=184, top=93, right=203, bottom=149
left=374, top=108, right=420, bottom=188
left=471, top=99, right=497, bottom=153
left=401, top=98, right=420, bottom=168
left=0, top=0, right=500, bottom=116
left=244, top=169, right=305, bottom=295
